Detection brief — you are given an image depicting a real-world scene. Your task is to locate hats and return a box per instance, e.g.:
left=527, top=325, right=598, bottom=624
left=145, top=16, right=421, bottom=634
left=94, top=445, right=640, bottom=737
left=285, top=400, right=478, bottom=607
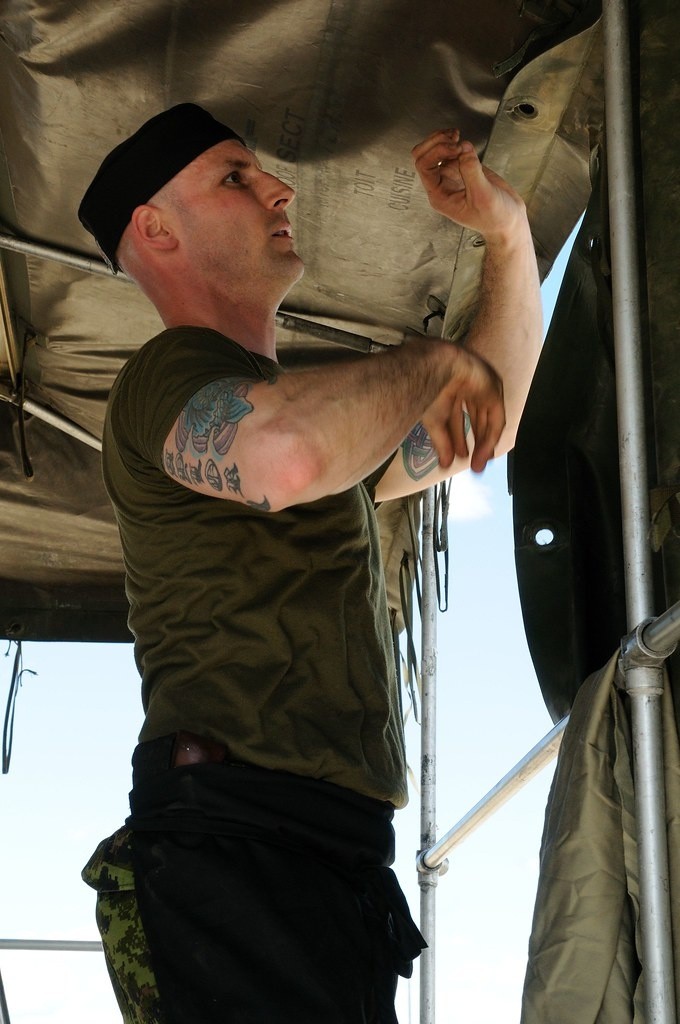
left=78, top=103, right=246, bottom=274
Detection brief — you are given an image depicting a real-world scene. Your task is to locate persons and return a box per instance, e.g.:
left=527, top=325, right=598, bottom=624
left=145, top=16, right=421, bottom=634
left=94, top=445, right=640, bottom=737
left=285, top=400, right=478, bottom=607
left=76, top=101, right=543, bottom=1024
